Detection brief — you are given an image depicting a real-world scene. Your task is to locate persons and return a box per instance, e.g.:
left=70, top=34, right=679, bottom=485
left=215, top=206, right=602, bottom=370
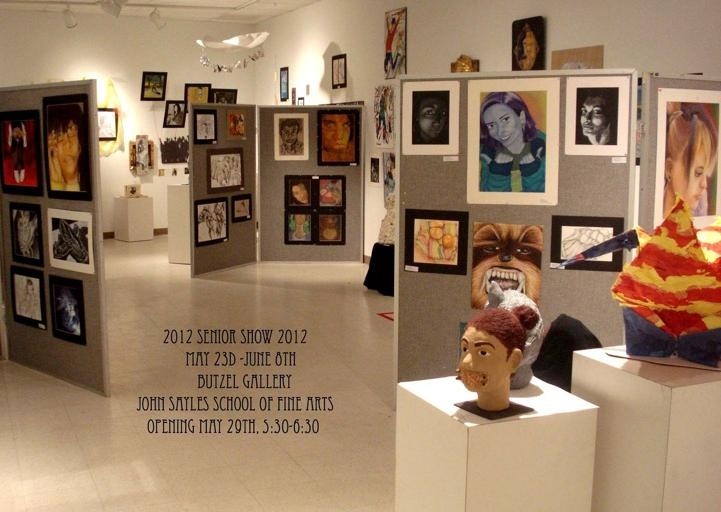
left=322, top=115, right=354, bottom=161
left=415, top=92, right=448, bottom=144
left=386, top=10, right=400, bottom=74
left=8, top=121, right=28, bottom=183
left=167, top=105, right=182, bottom=126
left=668, top=102, right=717, bottom=218
left=578, top=88, right=618, bottom=144
left=453, top=304, right=539, bottom=411
left=278, top=119, right=304, bottom=157
left=46, top=104, right=81, bottom=191
left=292, top=181, right=311, bottom=204
left=481, top=93, right=545, bottom=192
left=389, top=29, right=405, bottom=78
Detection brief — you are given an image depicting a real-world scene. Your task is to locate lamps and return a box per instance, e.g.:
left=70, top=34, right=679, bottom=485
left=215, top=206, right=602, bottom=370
left=63, top=4, right=80, bottom=29
left=99, top=0, right=124, bottom=19
left=149, top=7, right=167, bottom=32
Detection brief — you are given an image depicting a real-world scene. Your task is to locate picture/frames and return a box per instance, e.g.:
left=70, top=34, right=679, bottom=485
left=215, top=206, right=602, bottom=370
left=315, top=174, right=347, bottom=211
left=466, top=77, right=559, bottom=207
left=140, top=70, right=168, bottom=103
left=48, top=277, right=86, bottom=346
left=0, top=109, right=45, bottom=196
left=405, top=206, right=469, bottom=277
left=368, top=154, right=382, bottom=186
left=8, top=267, right=48, bottom=331
left=48, top=208, right=96, bottom=275
left=230, top=193, right=253, bottom=224
left=273, top=113, right=309, bottom=161
left=383, top=7, right=408, bottom=78
left=283, top=212, right=316, bottom=246
left=207, top=147, right=245, bottom=193
left=97, top=107, right=120, bottom=141
left=512, top=14, right=546, bottom=71
left=331, top=54, right=348, bottom=90
left=551, top=214, right=623, bottom=274
left=225, top=110, right=247, bottom=141
left=564, top=75, right=630, bottom=156
left=42, top=92, right=95, bottom=202
left=192, top=107, right=217, bottom=146
left=184, top=82, right=212, bottom=114
left=212, top=88, right=238, bottom=104
left=316, top=210, right=346, bottom=246
left=315, top=107, right=362, bottom=167
left=163, top=99, right=188, bottom=129
left=8, top=200, right=46, bottom=266
left=283, top=174, right=315, bottom=211
left=654, top=87, right=721, bottom=227
left=402, top=79, right=461, bottom=157
left=372, top=79, right=396, bottom=148
left=279, top=66, right=289, bottom=102
left=194, top=196, right=229, bottom=247
